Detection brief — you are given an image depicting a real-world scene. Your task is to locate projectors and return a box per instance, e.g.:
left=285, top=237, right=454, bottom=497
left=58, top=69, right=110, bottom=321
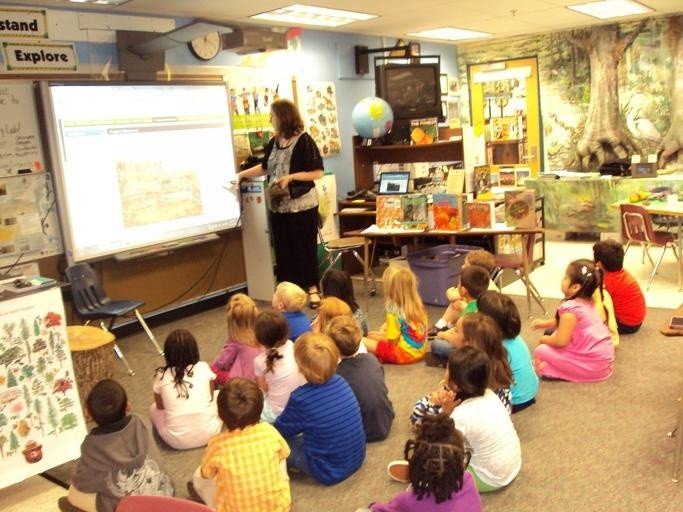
left=221, top=30, right=288, bottom=56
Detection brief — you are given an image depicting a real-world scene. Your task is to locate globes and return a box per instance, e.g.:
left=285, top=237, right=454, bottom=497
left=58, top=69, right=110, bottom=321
left=351, top=96, right=393, bottom=146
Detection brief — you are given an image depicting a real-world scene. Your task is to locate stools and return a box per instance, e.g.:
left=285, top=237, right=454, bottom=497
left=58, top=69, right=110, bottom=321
left=66, top=323, right=116, bottom=427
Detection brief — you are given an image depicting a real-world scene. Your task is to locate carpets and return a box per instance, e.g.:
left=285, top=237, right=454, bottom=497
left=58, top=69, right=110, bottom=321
left=42, top=275, right=683, bottom=512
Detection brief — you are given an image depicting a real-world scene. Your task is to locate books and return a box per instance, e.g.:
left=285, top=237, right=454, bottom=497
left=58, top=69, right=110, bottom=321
left=409, top=116, right=445, bottom=147
left=374, top=165, right=543, bottom=230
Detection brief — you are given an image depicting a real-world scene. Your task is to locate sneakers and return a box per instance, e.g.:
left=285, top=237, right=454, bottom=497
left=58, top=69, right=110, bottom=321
left=425, top=352, right=446, bottom=367
left=388, top=461, right=411, bottom=483
left=427, top=326, right=447, bottom=336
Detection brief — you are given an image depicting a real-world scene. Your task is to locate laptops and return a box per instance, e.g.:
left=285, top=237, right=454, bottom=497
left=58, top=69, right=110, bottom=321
left=366, top=171, right=410, bottom=200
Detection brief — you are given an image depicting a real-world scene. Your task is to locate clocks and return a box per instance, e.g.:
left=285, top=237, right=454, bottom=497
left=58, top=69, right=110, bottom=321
left=189, top=31, right=222, bottom=60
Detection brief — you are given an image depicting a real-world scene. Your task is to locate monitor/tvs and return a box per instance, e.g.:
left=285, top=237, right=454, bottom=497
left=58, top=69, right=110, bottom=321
left=376, top=63, right=442, bottom=119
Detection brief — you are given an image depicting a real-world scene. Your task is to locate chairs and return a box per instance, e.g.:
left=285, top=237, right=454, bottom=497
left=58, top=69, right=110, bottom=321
left=620, top=204, right=683, bottom=292
left=65, top=262, right=166, bottom=377
left=116, top=496, right=215, bottom=512
left=485, top=232, right=548, bottom=317
left=317, top=212, right=376, bottom=296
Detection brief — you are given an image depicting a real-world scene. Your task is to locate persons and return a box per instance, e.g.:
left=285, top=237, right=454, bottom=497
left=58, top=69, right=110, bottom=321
left=184, top=377, right=295, bottom=511
left=272, top=334, right=369, bottom=487
left=230, top=100, right=326, bottom=310
left=228, top=85, right=282, bottom=115
left=149, top=330, right=227, bottom=450
left=54, top=378, right=173, bottom=512
left=361, top=414, right=491, bottom=512
left=216, top=269, right=395, bottom=443
left=531, top=240, right=645, bottom=382
left=364, top=250, right=540, bottom=490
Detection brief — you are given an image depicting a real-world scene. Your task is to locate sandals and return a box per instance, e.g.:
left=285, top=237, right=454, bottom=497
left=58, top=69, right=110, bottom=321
left=309, top=290, right=321, bottom=308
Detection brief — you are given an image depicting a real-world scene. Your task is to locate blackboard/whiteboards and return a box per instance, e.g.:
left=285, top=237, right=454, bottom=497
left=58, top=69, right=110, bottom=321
left=0, top=80, right=63, bottom=269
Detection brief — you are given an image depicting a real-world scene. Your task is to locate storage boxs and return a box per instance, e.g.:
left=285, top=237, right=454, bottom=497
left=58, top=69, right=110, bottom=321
left=408, top=244, right=484, bottom=306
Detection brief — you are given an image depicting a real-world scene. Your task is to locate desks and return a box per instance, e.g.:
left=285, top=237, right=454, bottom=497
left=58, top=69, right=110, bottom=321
left=0, top=278, right=88, bottom=490
left=342, top=224, right=545, bottom=306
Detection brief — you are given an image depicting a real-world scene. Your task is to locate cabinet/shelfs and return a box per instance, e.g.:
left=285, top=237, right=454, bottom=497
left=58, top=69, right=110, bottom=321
left=338, top=127, right=547, bottom=278
left=486, top=139, right=526, bottom=165
